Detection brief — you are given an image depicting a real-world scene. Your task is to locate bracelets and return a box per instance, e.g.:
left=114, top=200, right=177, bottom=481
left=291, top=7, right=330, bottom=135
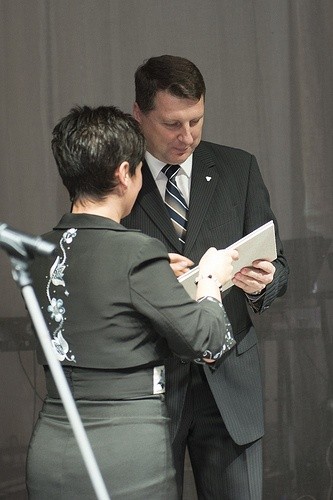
left=252, top=284, right=266, bottom=296
left=194, top=273, right=223, bottom=292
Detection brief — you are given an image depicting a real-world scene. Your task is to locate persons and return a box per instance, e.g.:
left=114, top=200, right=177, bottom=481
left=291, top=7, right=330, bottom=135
left=23, top=106, right=240, bottom=500
left=115, top=53, right=291, bottom=500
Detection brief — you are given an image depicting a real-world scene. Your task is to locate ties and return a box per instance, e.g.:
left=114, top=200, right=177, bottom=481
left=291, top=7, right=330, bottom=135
left=161, top=163, right=189, bottom=249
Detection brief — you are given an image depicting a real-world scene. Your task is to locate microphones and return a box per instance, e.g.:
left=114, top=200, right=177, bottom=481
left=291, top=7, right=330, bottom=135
left=0, top=223, right=57, bottom=257
